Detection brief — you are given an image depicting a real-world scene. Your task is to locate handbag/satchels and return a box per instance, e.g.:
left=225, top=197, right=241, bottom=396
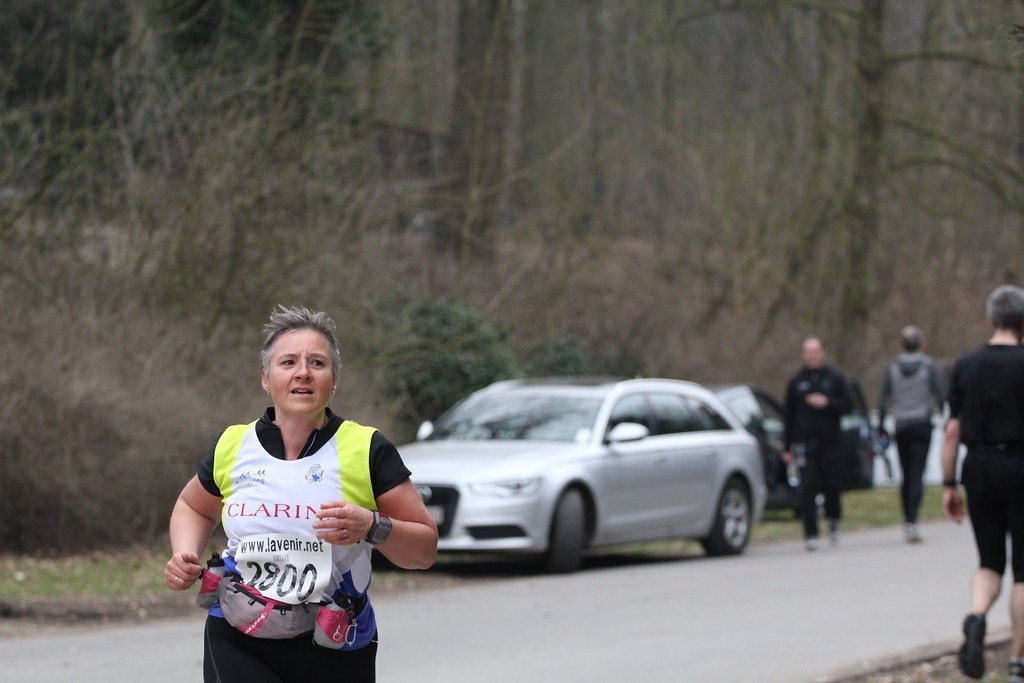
left=838, top=426, right=874, bottom=489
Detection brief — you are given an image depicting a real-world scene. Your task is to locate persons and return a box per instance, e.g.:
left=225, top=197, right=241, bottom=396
left=165, top=307, right=437, bottom=682
left=782, top=338, right=854, bottom=549
left=942, top=286, right=1024, bottom=679
left=879, top=325, right=947, bottom=542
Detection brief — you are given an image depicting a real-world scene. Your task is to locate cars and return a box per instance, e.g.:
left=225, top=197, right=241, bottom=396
left=709, top=380, right=969, bottom=511
left=370, top=373, right=770, bottom=576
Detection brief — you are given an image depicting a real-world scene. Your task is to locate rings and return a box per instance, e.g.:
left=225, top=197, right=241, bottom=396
left=174, top=577, right=179, bottom=584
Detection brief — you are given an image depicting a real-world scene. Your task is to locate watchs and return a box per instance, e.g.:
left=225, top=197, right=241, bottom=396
left=357, top=510, right=393, bottom=545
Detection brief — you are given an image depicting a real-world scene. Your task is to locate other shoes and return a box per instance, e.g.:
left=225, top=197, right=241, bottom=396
left=959, top=614, right=985, bottom=678
left=905, top=523, right=920, bottom=543
left=1009, top=657, right=1024, bottom=683
left=806, top=538, right=820, bottom=551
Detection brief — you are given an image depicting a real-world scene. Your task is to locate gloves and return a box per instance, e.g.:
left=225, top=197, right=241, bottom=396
left=878, top=429, right=889, bottom=450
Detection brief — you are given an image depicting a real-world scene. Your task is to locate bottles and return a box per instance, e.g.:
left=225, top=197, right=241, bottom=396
left=207, top=552, right=226, bottom=578
left=326, top=589, right=351, bottom=611
left=787, top=459, right=800, bottom=486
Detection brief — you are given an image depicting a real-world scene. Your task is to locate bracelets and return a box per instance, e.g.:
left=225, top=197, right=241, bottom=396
left=942, top=479, right=958, bottom=488
